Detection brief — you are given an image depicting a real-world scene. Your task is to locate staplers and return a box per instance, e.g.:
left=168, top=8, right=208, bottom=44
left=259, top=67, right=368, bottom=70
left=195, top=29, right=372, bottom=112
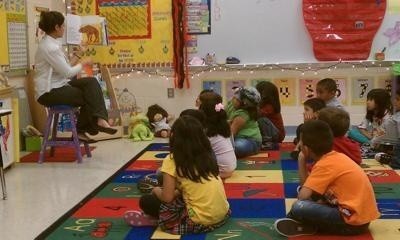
left=226, top=57, right=240, bottom=64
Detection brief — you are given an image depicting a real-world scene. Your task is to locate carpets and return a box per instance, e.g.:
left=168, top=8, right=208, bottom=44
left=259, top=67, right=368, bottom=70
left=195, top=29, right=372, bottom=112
left=17, top=144, right=98, bottom=162
left=33, top=142, right=400, bottom=239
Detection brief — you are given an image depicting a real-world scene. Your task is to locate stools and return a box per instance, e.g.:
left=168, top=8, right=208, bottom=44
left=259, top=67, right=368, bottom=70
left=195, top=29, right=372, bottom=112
left=36, top=106, right=92, bottom=162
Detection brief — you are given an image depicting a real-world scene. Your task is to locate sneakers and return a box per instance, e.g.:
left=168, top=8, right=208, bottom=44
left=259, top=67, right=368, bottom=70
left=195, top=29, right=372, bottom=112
left=275, top=219, right=318, bottom=237
left=144, top=174, right=164, bottom=186
left=124, top=211, right=159, bottom=226
left=375, top=153, right=392, bottom=164
left=137, top=183, right=156, bottom=193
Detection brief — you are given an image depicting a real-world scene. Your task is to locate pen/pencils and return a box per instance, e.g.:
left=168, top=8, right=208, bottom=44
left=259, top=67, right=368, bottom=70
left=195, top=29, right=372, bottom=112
left=208, top=51, right=215, bottom=56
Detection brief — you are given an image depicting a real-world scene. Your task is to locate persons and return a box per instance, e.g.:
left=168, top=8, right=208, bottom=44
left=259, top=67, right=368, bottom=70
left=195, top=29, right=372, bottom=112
left=32, top=11, right=118, bottom=136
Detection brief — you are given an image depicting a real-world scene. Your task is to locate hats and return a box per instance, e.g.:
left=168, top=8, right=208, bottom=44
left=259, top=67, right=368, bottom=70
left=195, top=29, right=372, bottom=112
left=233, top=85, right=261, bottom=110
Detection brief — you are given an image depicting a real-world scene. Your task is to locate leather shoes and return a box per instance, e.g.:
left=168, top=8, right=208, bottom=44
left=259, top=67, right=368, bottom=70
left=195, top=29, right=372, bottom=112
left=70, top=136, right=98, bottom=144
left=91, top=125, right=118, bottom=134
